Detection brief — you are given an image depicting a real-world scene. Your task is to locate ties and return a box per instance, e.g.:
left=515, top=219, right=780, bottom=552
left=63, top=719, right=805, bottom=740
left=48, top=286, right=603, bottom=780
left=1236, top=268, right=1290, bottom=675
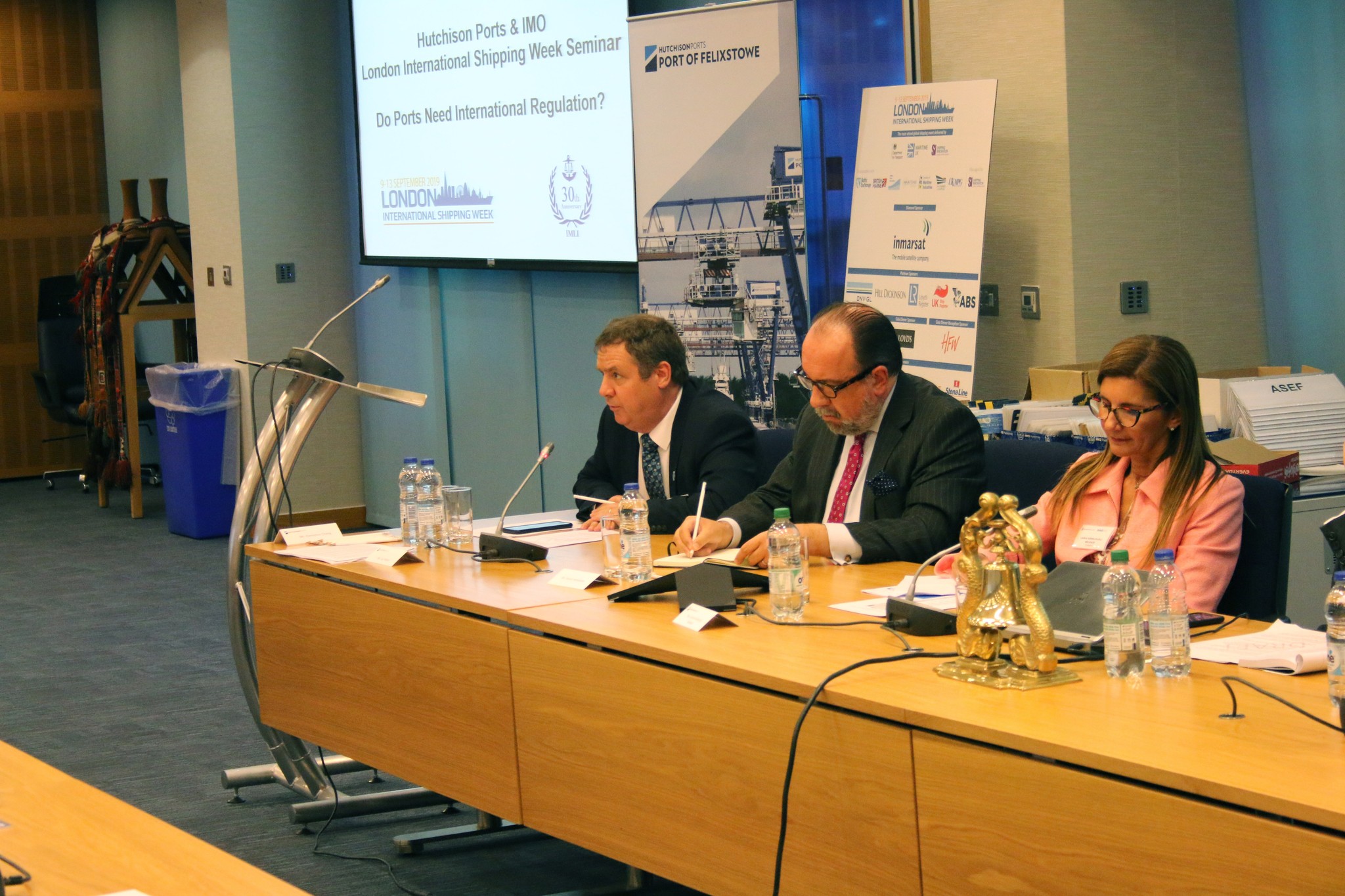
left=641, top=433, right=668, bottom=500
left=827, top=434, right=867, bottom=524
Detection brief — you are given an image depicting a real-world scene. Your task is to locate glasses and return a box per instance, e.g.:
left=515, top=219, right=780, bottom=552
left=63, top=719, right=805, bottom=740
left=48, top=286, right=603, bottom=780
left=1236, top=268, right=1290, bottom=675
left=794, top=363, right=887, bottom=398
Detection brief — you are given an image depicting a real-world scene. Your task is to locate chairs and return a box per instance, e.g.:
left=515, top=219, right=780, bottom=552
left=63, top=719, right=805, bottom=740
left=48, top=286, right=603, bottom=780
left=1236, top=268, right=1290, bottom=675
left=33, top=276, right=162, bottom=493
left=984, top=439, right=1292, bottom=624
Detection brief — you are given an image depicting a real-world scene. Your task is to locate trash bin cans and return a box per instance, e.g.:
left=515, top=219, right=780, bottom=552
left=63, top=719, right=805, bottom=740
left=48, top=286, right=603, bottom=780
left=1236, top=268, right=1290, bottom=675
left=146, top=364, right=240, bottom=538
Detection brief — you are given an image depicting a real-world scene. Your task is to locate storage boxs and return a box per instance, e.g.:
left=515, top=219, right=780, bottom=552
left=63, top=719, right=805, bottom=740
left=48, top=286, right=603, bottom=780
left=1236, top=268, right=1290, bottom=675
left=1220, top=451, right=1300, bottom=491
left=1197, top=365, right=1323, bottom=429
left=1029, top=360, right=1101, bottom=400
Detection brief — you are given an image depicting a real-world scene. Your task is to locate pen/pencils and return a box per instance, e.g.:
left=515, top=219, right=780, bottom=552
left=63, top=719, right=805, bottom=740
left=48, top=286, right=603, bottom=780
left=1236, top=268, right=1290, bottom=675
left=1054, top=647, right=1091, bottom=655
left=574, top=495, right=615, bottom=504
left=690, top=482, right=707, bottom=560
left=1067, top=639, right=1104, bottom=649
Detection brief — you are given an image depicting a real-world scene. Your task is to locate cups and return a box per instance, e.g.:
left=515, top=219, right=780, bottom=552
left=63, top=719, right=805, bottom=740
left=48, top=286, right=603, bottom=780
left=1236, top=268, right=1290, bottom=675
left=441, top=485, right=473, bottom=544
left=601, top=514, right=619, bottom=569
left=800, top=536, right=811, bottom=604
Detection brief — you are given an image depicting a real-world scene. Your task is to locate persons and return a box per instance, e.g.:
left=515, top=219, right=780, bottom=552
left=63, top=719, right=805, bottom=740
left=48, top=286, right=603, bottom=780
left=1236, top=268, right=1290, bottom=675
left=672, top=301, right=986, bottom=567
left=933, top=336, right=1244, bottom=614
left=573, top=313, right=757, bottom=532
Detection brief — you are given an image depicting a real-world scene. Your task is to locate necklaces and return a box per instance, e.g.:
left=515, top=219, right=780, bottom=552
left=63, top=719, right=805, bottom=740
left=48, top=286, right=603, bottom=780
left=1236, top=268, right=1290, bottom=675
left=1131, top=474, right=1150, bottom=489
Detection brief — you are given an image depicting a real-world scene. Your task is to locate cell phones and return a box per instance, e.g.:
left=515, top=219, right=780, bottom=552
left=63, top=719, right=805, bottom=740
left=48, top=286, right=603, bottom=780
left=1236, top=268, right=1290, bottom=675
left=1186, top=612, right=1225, bottom=627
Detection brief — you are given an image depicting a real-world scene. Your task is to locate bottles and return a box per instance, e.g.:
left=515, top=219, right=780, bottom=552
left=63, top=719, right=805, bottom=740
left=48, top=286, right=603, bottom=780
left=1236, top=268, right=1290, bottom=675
left=1324, top=571, right=1345, bottom=707
left=1146, top=549, right=1192, bottom=678
left=619, top=483, right=653, bottom=583
left=399, top=457, right=419, bottom=546
left=1101, top=550, right=1145, bottom=680
left=767, top=507, right=806, bottom=622
left=415, top=458, right=445, bottom=549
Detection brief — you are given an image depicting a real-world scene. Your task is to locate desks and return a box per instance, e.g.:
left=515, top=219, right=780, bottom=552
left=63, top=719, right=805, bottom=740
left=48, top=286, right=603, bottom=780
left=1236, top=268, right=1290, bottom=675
left=0, top=741, right=310, bottom=896
left=244, top=526, right=1345, bottom=896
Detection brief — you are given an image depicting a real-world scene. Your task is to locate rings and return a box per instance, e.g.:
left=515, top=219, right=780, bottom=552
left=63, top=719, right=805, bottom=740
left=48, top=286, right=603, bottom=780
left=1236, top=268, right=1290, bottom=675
left=609, top=509, right=611, bottom=513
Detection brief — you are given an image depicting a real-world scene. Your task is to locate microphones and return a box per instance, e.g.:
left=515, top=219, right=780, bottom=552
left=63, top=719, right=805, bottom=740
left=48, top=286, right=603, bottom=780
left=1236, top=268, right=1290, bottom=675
left=884, top=508, right=1036, bottom=638
left=285, top=275, right=394, bottom=381
left=479, top=443, right=555, bottom=560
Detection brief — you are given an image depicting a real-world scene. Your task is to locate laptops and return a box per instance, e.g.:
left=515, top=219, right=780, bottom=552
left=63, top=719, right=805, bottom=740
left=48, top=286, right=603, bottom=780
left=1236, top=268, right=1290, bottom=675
left=1037, top=560, right=1153, bottom=642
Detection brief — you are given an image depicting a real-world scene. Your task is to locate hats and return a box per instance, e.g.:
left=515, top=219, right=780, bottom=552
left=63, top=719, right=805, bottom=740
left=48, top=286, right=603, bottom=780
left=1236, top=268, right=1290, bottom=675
left=1088, top=391, right=1169, bottom=427
